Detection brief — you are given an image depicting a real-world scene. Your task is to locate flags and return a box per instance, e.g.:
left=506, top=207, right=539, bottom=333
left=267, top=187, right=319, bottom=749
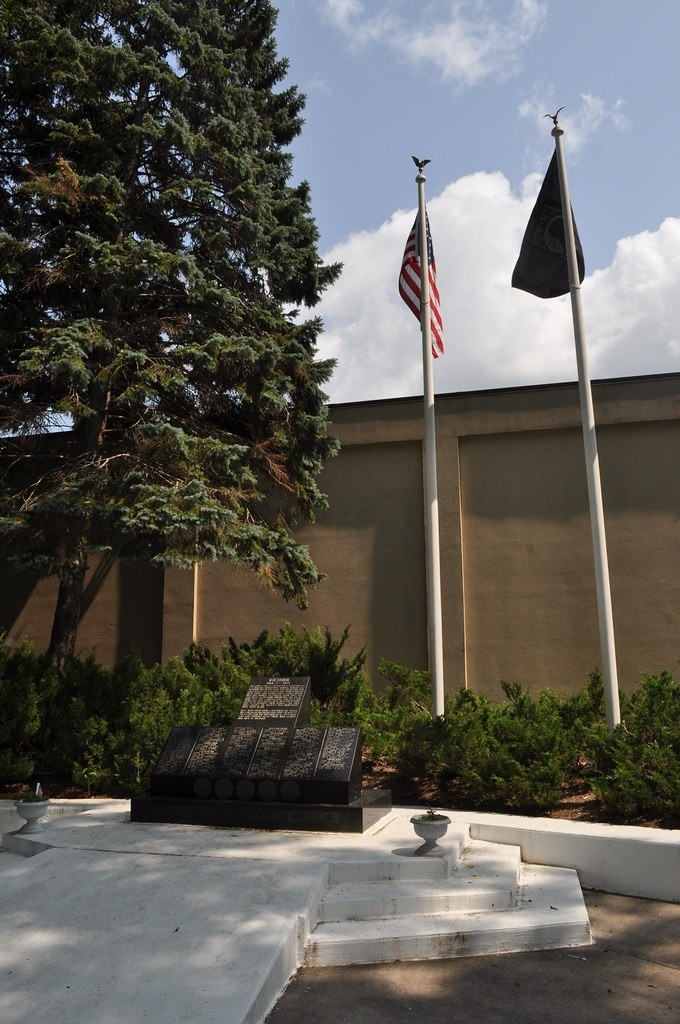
left=398, top=207, right=444, bottom=359
left=512, top=147, right=584, bottom=299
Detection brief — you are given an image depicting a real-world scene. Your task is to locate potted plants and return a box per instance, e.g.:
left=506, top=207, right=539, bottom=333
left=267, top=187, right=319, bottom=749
left=13, top=786, right=50, bottom=834
left=410, top=800, right=451, bottom=856
